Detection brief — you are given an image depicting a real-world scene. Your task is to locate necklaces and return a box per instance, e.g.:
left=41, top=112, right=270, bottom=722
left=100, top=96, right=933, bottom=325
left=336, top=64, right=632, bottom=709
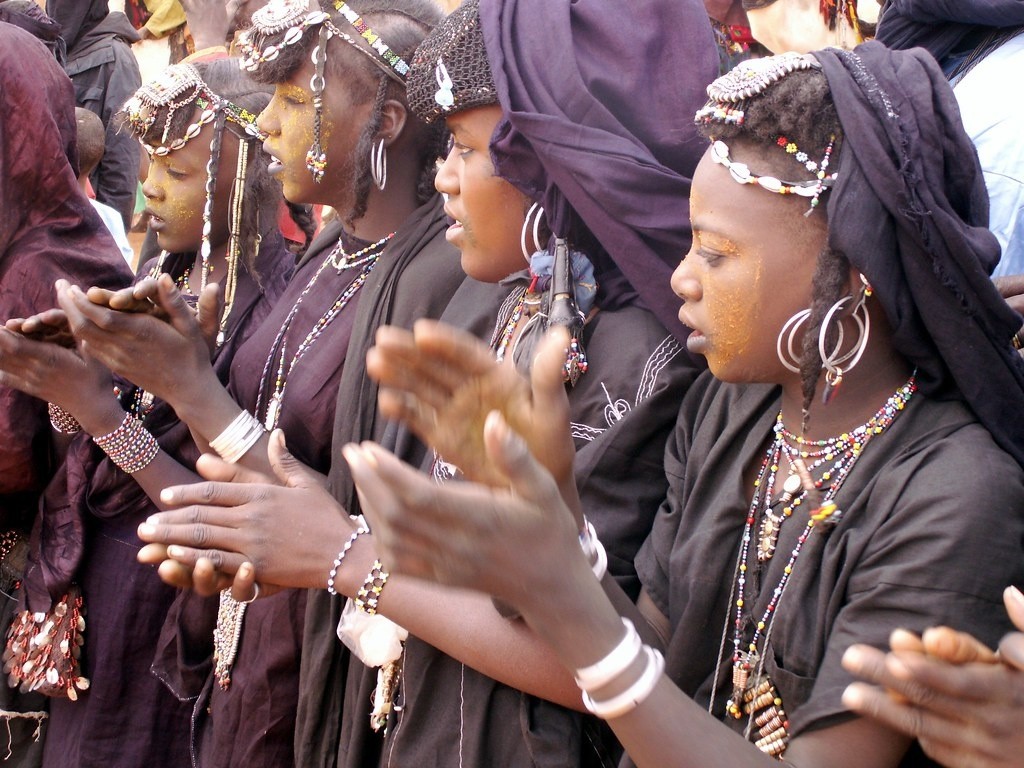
left=44, top=401, right=80, bottom=435
left=93, top=411, right=160, bottom=476
left=494, top=283, right=528, bottom=371
left=130, top=266, right=215, bottom=422
left=725, top=378, right=927, bottom=743
left=252, top=229, right=402, bottom=439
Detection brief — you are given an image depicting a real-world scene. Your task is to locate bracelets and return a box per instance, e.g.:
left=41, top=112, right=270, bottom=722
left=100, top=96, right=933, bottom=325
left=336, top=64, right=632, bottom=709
left=578, top=516, right=598, bottom=558
left=328, top=526, right=365, bottom=595
left=355, top=554, right=392, bottom=617
left=568, top=611, right=668, bottom=720
left=586, top=540, right=610, bottom=584
left=206, top=408, right=266, bottom=468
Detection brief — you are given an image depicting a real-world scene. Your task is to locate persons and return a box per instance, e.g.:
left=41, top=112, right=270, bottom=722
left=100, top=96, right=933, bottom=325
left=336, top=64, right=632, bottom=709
left=838, top=581, right=1024, bottom=767
left=1, top=47, right=317, bottom=768
left=104, top=0, right=188, bottom=89
left=50, top=1, right=449, bottom=768
left=43, top=0, right=143, bottom=242
left=337, top=35, right=1024, bottom=768
left=744, top=0, right=868, bottom=67
left=871, top=1, right=1024, bottom=365
left=62, top=105, right=137, bottom=272
left=2, top=19, right=130, bottom=768
left=134, top=2, right=745, bottom=767
